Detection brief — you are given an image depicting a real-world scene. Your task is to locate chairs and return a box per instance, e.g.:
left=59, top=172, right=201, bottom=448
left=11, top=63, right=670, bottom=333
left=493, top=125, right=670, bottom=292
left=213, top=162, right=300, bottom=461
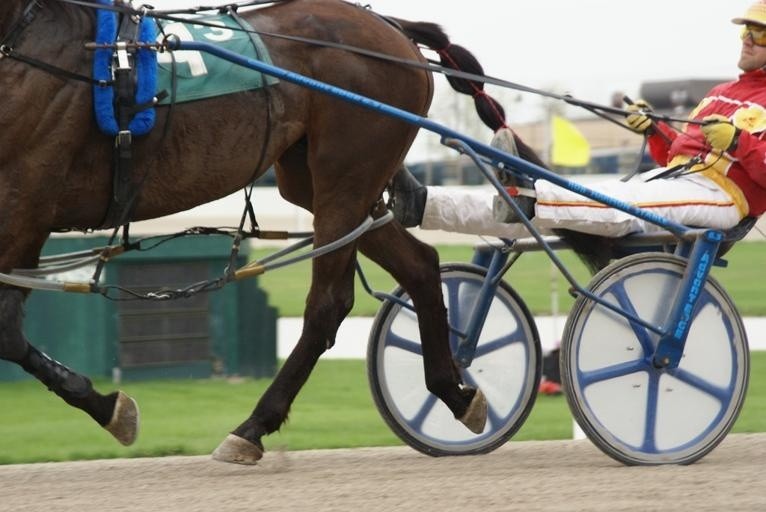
left=509, top=155, right=759, bottom=265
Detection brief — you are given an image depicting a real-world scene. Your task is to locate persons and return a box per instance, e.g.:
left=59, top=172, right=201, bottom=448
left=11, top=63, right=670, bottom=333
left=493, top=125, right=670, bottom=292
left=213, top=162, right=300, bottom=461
left=388, top=0, right=766, bottom=240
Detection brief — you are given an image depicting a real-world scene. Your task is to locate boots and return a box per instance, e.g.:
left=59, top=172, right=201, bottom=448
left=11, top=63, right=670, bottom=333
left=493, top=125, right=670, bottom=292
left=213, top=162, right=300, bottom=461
left=489, top=128, right=537, bottom=224
left=392, top=163, right=427, bottom=228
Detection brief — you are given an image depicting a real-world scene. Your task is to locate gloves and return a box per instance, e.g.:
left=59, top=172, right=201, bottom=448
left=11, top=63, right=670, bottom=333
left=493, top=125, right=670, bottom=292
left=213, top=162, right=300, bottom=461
left=701, top=113, right=736, bottom=152
left=625, top=99, right=655, bottom=133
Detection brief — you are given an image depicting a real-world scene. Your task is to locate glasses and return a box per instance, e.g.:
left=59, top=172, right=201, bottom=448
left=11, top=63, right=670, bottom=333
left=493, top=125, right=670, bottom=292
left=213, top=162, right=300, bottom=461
left=738, top=25, right=766, bottom=47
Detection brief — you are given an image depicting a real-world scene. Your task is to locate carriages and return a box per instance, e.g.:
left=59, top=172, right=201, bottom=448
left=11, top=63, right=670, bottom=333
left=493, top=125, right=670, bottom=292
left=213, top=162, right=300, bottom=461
left=0, top=1, right=766, bottom=466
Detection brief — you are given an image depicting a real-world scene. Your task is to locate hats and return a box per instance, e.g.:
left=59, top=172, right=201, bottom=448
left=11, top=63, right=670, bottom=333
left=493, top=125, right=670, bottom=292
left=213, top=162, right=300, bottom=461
left=731, top=0, right=766, bottom=25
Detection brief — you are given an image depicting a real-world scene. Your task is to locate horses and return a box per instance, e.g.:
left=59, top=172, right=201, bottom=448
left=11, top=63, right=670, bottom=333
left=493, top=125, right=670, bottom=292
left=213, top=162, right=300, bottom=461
left=0, top=0, right=552, bottom=467
